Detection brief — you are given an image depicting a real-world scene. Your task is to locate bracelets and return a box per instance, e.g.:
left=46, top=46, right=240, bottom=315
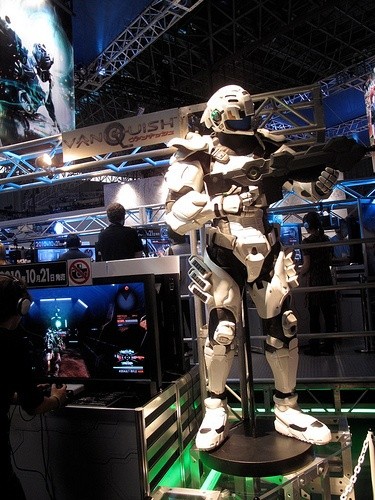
left=49, top=394, right=61, bottom=408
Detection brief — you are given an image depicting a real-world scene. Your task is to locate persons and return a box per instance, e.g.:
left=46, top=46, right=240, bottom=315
left=97, top=202, right=143, bottom=284
left=330, top=218, right=350, bottom=267
left=164, top=84, right=339, bottom=451
left=158, top=226, right=199, bottom=367
left=58, top=234, right=91, bottom=261
left=295, top=211, right=336, bottom=357
left=0, top=242, right=12, bottom=265
left=0, top=273, right=69, bottom=500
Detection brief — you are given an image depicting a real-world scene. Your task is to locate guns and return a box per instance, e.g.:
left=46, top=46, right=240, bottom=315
left=202, top=135, right=375, bottom=206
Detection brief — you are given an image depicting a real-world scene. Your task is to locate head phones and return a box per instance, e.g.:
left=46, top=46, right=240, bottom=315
left=63, top=234, right=82, bottom=248
left=0, top=274, right=33, bottom=317
left=303, top=214, right=322, bottom=229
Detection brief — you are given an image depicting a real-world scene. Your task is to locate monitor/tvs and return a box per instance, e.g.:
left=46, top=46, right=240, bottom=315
left=34, top=239, right=60, bottom=246
left=155, top=273, right=185, bottom=368
left=279, top=223, right=303, bottom=263
left=26, top=273, right=162, bottom=388
left=34, top=245, right=96, bottom=263
left=141, top=237, right=173, bottom=258
left=346, top=216, right=364, bottom=265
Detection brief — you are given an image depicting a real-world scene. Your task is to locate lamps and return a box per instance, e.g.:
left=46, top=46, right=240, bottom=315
left=35, top=153, right=52, bottom=178
